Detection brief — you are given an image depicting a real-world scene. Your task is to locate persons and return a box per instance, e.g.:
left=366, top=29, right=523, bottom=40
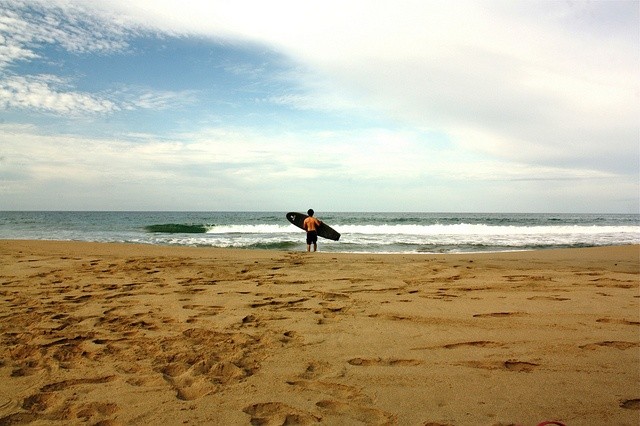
left=303, top=209, right=321, bottom=252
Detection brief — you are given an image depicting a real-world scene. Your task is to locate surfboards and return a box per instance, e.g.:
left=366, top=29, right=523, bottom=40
left=286, top=212, right=341, bottom=242
